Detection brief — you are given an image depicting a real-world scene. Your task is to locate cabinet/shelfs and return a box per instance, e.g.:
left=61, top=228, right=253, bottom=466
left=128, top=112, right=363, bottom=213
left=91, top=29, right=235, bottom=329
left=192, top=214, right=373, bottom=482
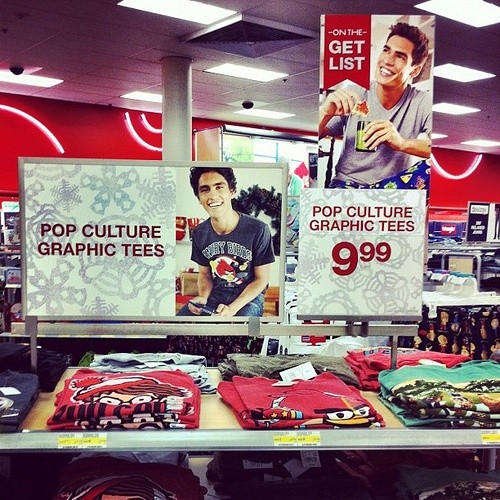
left=0, top=124, right=500, bottom=500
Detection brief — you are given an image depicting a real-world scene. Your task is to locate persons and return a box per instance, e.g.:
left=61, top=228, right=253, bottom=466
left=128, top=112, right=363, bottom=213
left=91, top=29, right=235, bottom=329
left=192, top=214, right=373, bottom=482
left=318, top=23, right=432, bottom=207
left=176, top=167, right=275, bottom=316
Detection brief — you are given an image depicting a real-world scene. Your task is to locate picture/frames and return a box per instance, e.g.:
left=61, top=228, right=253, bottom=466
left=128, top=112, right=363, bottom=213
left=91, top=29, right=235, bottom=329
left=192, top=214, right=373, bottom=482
left=19, top=155, right=287, bottom=323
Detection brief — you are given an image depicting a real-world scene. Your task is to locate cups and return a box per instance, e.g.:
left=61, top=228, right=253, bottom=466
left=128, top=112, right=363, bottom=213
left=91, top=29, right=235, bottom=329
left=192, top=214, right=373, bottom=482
left=355, top=121, right=377, bottom=152
